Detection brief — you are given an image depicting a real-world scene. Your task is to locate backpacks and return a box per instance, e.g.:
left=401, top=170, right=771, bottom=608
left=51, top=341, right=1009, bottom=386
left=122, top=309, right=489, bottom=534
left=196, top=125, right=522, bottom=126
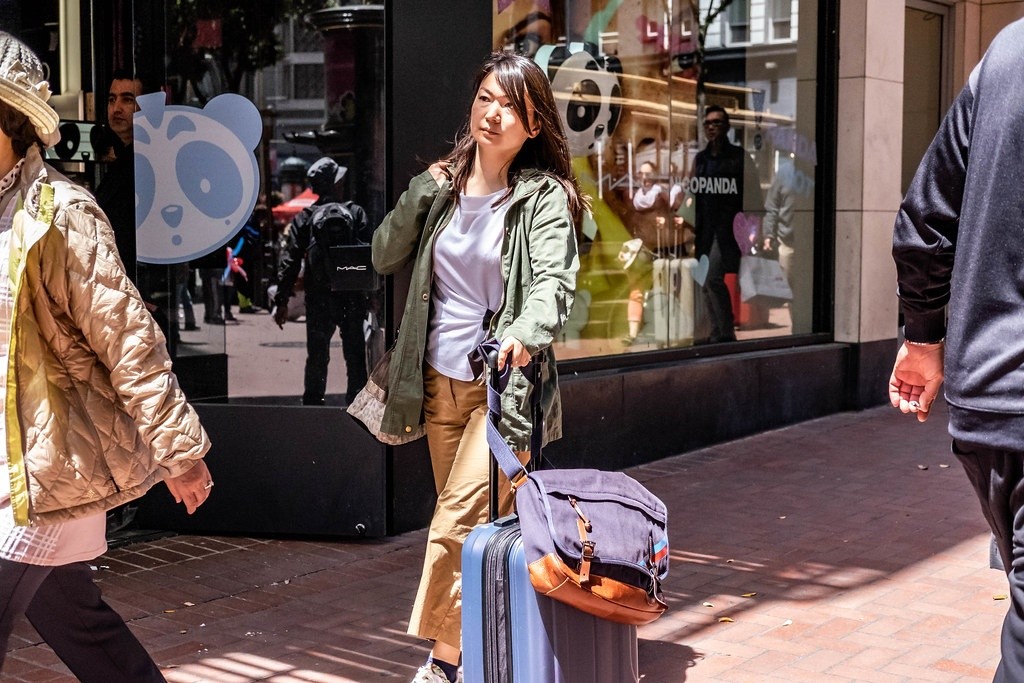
left=302, top=199, right=366, bottom=293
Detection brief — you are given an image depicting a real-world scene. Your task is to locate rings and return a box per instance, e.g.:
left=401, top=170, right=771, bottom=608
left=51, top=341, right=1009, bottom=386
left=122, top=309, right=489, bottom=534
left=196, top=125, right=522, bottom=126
left=204, top=480, right=214, bottom=489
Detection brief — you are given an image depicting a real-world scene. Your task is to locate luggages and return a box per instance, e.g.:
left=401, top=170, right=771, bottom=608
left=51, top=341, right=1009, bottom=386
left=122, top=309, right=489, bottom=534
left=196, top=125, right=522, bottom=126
left=653, top=216, right=670, bottom=349
left=459, top=351, right=642, bottom=683
left=669, top=217, right=710, bottom=349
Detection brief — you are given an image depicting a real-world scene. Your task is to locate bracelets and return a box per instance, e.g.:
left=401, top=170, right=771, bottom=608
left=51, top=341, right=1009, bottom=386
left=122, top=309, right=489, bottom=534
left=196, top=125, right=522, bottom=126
left=904, top=336, right=945, bottom=346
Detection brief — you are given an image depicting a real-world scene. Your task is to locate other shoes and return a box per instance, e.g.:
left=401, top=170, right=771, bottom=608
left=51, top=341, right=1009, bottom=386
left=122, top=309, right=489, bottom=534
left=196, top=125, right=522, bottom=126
left=621, top=335, right=636, bottom=345
left=239, top=305, right=262, bottom=314
left=184, top=325, right=201, bottom=331
left=411, top=662, right=463, bottom=683
left=204, top=317, right=224, bottom=325
left=223, top=314, right=237, bottom=322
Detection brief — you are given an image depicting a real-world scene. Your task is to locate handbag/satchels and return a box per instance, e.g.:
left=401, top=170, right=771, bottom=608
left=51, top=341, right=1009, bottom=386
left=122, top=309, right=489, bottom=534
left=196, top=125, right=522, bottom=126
left=739, top=248, right=793, bottom=310
left=513, top=469, right=669, bottom=626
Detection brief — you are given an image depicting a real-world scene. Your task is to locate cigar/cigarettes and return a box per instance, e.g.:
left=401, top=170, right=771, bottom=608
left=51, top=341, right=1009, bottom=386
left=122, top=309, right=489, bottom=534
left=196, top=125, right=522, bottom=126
left=910, top=401, right=921, bottom=410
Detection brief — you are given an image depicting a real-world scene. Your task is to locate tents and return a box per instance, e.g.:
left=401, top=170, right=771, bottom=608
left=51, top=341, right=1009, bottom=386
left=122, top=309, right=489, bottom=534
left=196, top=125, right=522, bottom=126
left=271, top=188, right=319, bottom=221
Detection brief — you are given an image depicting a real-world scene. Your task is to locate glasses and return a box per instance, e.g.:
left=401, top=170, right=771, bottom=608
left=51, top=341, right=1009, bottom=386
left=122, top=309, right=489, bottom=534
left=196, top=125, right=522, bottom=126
left=703, top=120, right=726, bottom=128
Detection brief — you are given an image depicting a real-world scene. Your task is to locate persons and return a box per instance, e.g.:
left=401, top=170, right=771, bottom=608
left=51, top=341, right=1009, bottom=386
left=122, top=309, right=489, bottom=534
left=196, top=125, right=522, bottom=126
left=371, top=54, right=591, bottom=683
left=888, top=17, right=1024, bottom=683
left=176, top=158, right=376, bottom=408
left=0, top=32, right=214, bottom=683
left=620, top=104, right=794, bottom=345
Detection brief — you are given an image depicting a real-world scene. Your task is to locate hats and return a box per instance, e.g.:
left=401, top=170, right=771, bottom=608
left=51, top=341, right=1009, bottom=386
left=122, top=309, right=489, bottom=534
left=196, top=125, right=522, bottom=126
left=307, top=156, right=348, bottom=193
left=0, top=31, right=62, bottom=149
